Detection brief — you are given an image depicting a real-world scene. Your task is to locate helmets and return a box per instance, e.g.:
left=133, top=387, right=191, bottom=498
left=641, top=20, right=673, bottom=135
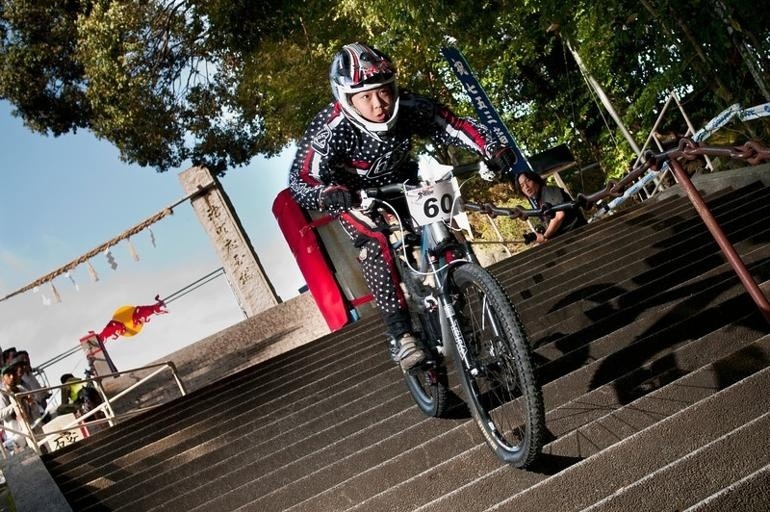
left=326, top=41, right=401, bottom=133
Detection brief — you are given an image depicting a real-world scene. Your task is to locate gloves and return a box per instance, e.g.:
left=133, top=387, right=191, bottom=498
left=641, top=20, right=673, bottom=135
left=320, top=182, right=352, bottom=210
left=486, top=144, right=519, bottom=173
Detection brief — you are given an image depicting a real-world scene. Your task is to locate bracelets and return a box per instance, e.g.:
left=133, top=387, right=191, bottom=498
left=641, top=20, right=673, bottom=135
left=542, top=234, right=549, bottom=242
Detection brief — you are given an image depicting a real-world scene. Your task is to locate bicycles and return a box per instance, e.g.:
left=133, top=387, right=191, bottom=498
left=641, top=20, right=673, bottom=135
left=354, top=159, right=545, bottom=471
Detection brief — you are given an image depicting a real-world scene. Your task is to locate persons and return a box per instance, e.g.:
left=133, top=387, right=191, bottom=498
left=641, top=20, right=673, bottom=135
left=287, top=40, right=518, bottom=376
left=8, top=350, right=53, bottom=423
left=0, top=363, right=44, bottom=451
left=516, top=171, right=589, bottom=247
left=0, top=347, right=20, bottom=369
left=61, top=373, right=104, bottom=415
left=8, top=358, right=40, bottom=422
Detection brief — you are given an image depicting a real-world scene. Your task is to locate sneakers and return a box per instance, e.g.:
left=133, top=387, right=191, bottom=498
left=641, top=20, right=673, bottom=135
left=387, top=332, right=428, bottom=373
left=456, top=312, right=481, bottom=358
